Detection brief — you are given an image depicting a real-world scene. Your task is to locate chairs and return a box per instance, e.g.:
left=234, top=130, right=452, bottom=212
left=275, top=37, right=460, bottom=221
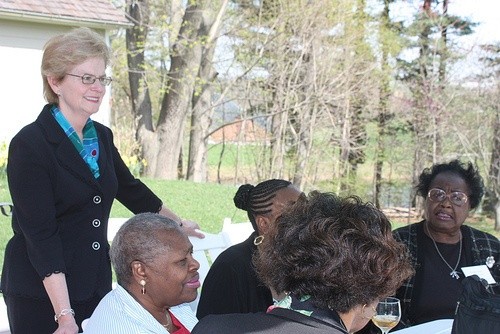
left=188, top=225, right=231, bottom=312
left=220, top=217, right=255, bottom=242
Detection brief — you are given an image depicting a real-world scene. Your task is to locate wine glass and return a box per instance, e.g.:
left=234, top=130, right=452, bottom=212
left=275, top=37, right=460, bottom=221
left=372, top=297, right=402, bottom=334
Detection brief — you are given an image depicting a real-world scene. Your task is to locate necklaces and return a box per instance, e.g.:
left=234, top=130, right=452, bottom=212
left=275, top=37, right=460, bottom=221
left=426, top=224, right=463, bottom=279
left=163, top=314, right=171, bottom=331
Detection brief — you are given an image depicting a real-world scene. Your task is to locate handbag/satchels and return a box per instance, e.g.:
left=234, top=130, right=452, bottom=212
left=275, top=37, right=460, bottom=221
left=448, top=275, right=500, bottom=334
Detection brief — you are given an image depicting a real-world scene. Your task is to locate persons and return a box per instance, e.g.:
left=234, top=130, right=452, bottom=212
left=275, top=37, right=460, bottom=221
left=191, top=191, right=415, bottom=334
left=355, top=159, right=500, bottom=334
left=0, top=25, right=205, bottom=334
left=196, top=179, right=301, bottom=316
left=82, top=212, right=200, bottom=334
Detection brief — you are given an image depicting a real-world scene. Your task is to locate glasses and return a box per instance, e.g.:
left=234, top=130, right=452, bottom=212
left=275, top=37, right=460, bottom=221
left=65, top=73, right=113, bottom=86
left=427, top=188, right=472, bottom=206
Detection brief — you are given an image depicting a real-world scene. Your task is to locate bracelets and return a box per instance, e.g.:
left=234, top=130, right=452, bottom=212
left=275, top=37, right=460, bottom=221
left=54, top=308, right=76, bottom=322
left=179, top=220, right=183, bottom=226
